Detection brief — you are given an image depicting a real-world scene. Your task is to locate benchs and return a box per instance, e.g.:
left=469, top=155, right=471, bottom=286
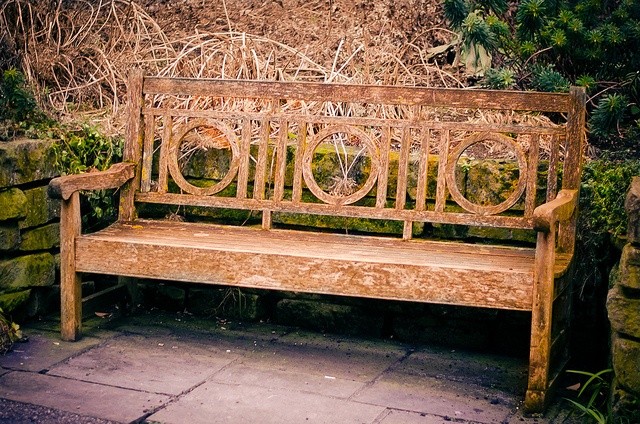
left=47, top=67, right=587, bottom=419
